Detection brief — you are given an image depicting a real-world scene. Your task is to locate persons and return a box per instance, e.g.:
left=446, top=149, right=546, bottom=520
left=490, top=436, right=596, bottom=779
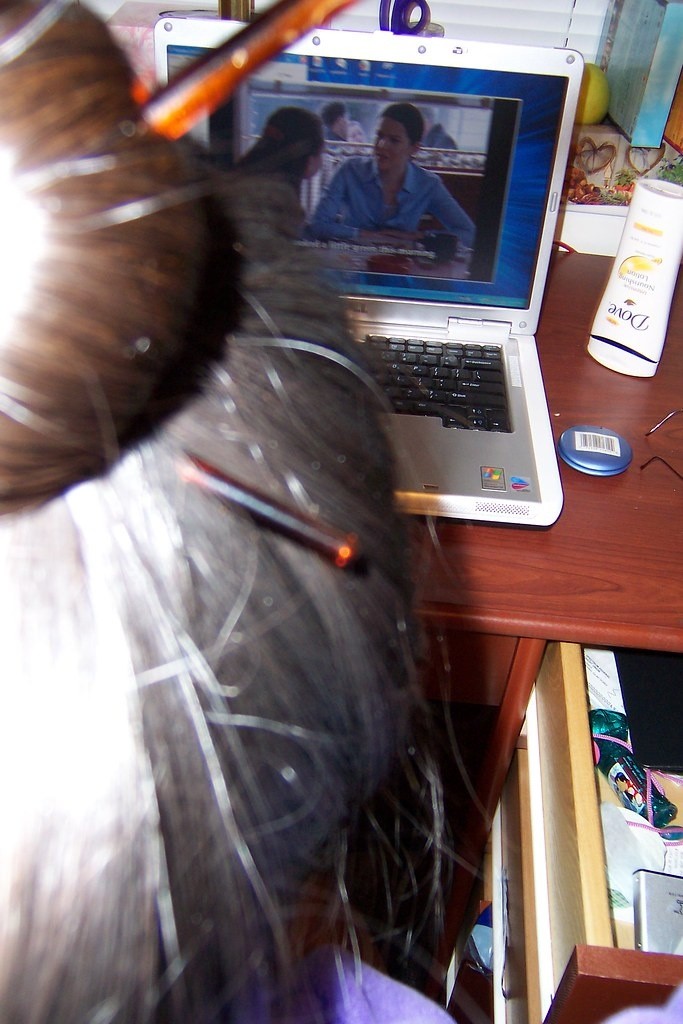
left=234, top=98, right=479, bottom=249
left=0, top=0, right=467, bottom=1024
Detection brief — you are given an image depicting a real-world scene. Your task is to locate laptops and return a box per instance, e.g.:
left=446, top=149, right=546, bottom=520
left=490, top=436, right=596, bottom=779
left=154, top=15, right=588, bottom=529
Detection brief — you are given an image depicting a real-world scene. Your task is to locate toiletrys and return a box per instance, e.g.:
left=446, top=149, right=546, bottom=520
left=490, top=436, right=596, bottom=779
left=587, top=179, right=683, bottom=378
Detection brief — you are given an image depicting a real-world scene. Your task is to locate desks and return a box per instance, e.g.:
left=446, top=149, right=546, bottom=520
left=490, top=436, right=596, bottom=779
left=418, top=250, right=683, bottom=1024
